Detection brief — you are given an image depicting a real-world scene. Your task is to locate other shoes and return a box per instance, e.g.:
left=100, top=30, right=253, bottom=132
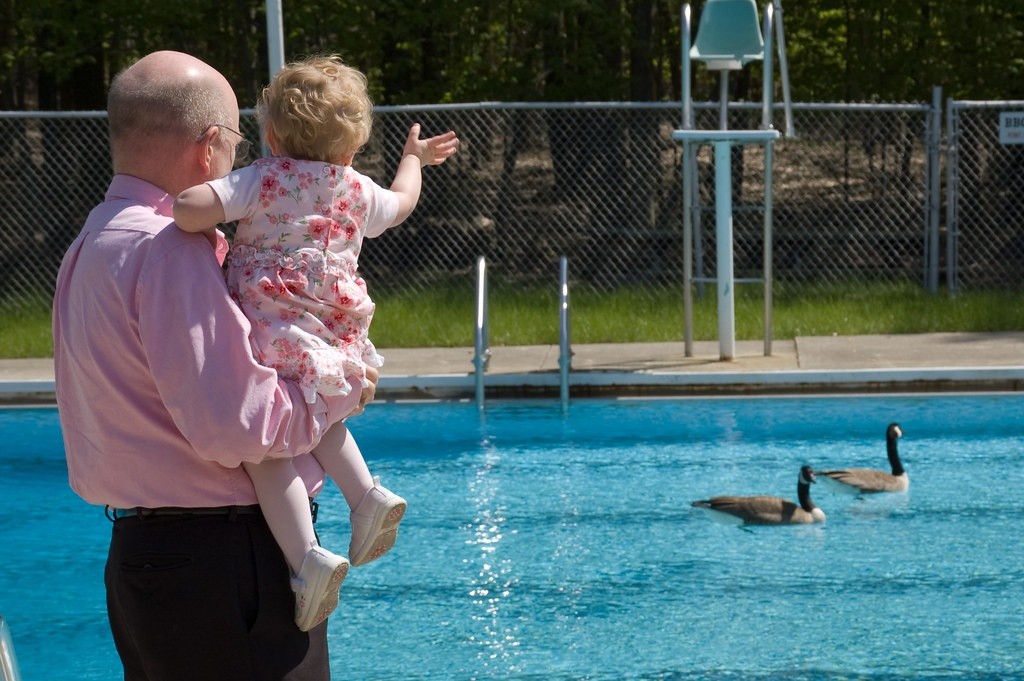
left=290, top=540, right=350, bottom=632
left=345, top=475, right=408, bottom=567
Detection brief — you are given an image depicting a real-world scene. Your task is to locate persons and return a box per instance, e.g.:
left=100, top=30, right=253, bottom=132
left=51, top=49, right=378, bottom=681
left=175, top=57, right=458, bottom=633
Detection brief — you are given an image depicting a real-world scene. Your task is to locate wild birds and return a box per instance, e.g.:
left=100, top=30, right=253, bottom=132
left=691, top=465, right=827, bottom=526
left=814, top=423, right=910, bottom=494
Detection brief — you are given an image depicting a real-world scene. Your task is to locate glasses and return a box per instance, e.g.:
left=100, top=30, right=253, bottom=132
left=196, top=121, right=255, bottom=161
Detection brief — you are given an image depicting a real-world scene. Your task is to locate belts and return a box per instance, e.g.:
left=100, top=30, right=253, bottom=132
left=111, top=501, right=262, bottom=519
